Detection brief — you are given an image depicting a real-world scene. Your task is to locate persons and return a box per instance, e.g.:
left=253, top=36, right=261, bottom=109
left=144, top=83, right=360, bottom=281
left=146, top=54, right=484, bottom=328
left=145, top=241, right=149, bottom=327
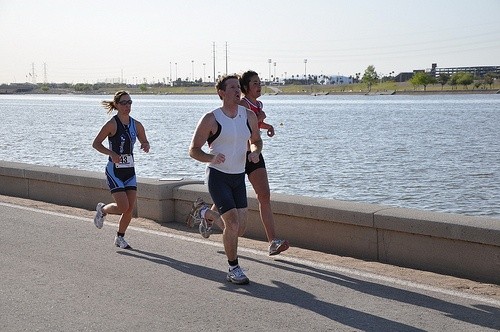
left=186, top=75, right=263, bottom=284
left=199, top=70, right=289, bottom=255
left=94, top=91, right=150, bottom=249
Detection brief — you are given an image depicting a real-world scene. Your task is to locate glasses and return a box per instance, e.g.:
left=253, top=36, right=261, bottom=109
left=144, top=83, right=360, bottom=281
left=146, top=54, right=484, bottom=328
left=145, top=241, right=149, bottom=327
left=117, top=100, right=132, bottom=105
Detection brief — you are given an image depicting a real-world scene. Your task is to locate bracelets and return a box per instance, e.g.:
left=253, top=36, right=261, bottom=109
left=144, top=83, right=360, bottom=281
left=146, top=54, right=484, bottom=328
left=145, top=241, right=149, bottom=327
left=144, top=141, right=149, bottom=143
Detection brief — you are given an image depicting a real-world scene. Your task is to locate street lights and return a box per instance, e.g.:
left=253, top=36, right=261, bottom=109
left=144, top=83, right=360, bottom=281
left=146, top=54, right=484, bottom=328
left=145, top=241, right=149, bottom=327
left=192, top=60, right=194, bottom=81
left=202, top=63, right=206, bottom=82
left=175, top=62, right=177, bottom=80
left=267, top=58, right=272, bottom=82
left=274, top=61, right=276, bottom=77
left=304, top=59, right=307, bottom=85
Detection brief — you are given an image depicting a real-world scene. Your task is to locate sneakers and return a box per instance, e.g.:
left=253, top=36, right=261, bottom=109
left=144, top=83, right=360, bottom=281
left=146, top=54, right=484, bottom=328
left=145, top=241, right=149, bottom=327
left=114, top=235, right=130, bottom=249
left=186, top=197, right=207, bottom=228
left=94, top=202, right=107, bottom=229
left=199, top=218, right=213, bottom=239
left=269, top=240, right=289, bottom=256
left=226, top=265, right=248, bottom=284
